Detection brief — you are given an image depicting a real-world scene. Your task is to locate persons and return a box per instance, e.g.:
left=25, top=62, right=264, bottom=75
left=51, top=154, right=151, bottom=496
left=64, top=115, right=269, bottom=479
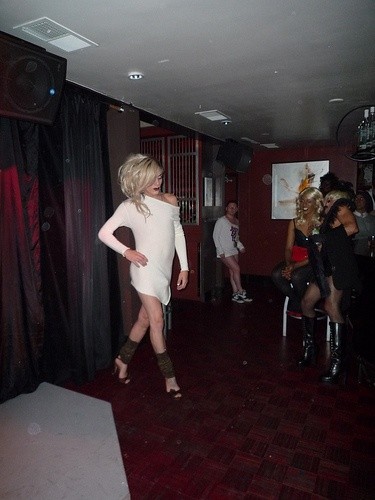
left=212, top=200, right=253, bottom=304
left=97, top=153, right=189, bottom=398
left=285, top=187, right=375, bottom=382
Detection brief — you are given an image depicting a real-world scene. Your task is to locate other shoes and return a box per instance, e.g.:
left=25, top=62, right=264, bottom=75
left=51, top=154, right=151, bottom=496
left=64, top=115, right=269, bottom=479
left=287, top=310, right=302, bottom=320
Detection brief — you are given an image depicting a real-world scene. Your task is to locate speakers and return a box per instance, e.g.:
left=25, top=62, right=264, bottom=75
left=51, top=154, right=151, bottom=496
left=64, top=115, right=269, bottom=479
left=0, top=31, right=69, bottom=125
left=217, top=138, right=255, bottom=173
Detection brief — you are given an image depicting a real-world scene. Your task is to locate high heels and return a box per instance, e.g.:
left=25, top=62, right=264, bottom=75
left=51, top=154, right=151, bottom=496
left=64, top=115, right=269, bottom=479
left=166, top=383, right=183, bottom=400
left=112, top=356, right=130, bottom=384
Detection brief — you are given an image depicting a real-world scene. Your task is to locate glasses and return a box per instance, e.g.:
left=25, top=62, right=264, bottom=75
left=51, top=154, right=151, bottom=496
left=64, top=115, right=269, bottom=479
left=354, top=197, right=365, bottom=201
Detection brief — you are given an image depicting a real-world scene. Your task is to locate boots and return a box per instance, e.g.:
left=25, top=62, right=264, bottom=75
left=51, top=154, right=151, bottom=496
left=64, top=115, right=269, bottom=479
left=297, top=314, right=317, bottom=366
left=321, top=322, right=348, bottom=386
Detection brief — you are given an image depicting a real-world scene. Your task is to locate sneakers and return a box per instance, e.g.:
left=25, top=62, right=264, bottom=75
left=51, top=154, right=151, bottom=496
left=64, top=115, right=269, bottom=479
left=237, top=290, right=254, bottom=302
left=232, top=292, right=244, bottom=303
